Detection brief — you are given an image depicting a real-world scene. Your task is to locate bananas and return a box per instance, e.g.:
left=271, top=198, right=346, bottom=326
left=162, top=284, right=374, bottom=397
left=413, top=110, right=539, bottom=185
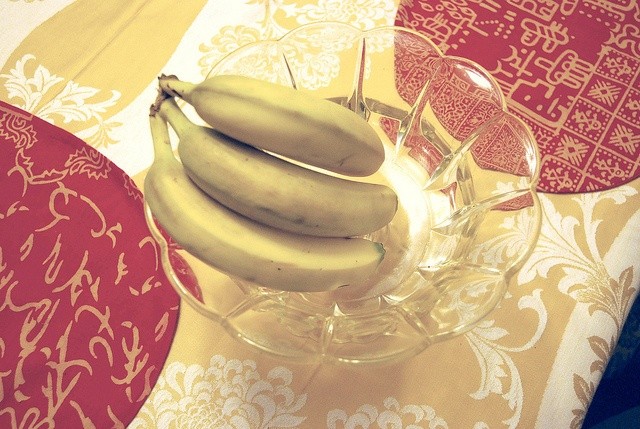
left=143, top=74, right=398, bottom=294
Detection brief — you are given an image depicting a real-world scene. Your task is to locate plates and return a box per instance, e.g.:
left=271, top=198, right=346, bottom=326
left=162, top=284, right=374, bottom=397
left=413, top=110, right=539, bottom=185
left=144, top=21, right=542, bottom=368
